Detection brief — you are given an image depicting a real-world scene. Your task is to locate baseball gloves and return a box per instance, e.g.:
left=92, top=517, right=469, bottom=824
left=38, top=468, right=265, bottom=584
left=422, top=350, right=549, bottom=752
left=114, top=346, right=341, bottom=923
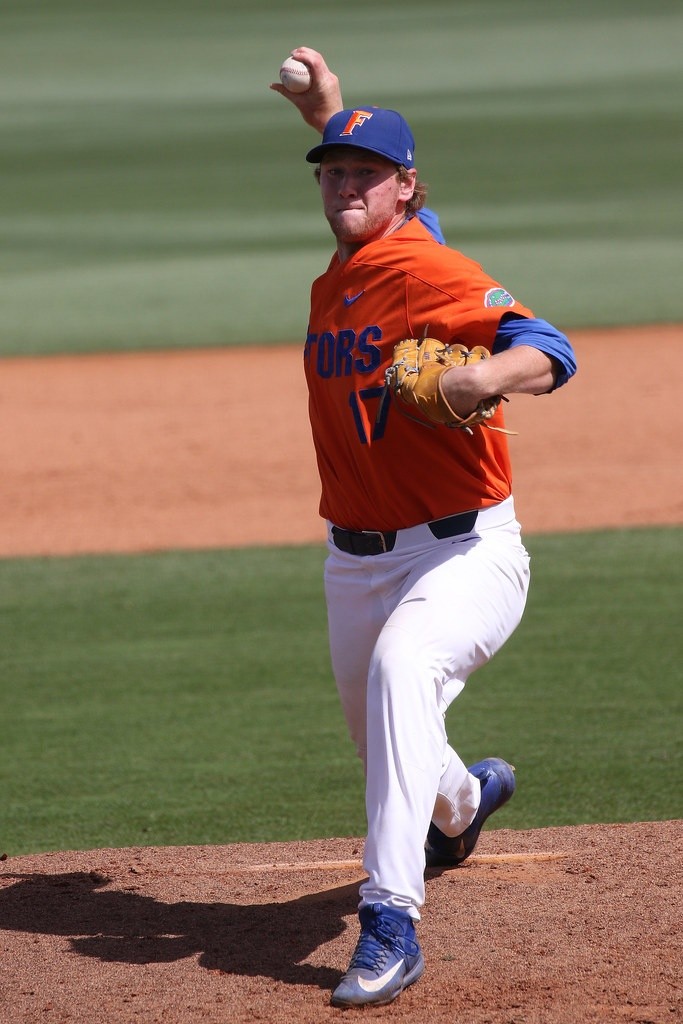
left=383, top=322, right=521, bottom=437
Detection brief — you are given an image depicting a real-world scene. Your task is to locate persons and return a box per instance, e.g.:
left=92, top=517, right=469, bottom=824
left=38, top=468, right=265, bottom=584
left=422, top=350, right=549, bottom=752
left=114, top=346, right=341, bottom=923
left=269, top=46, right=577, bottom=1009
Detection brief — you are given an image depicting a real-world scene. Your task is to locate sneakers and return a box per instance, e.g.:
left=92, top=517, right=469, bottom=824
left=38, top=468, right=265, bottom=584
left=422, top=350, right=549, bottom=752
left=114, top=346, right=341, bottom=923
left=424, top=757, right=515, bottom=868
left=329, top=902, right=425, bottom=1008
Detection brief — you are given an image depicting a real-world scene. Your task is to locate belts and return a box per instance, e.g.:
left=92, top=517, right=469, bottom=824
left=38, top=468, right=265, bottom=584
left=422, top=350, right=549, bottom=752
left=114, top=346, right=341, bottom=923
left=333, top=512, right=479, bottom=556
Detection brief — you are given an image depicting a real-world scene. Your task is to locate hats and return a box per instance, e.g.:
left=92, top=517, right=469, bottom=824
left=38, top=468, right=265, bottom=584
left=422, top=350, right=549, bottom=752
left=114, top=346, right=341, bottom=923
left=305, top=106, right=416, bottom=171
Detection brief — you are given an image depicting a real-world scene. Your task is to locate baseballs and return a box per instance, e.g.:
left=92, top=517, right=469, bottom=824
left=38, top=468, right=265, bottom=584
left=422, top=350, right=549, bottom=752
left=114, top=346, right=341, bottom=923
left=279, top=55, right=313, bottom=93
